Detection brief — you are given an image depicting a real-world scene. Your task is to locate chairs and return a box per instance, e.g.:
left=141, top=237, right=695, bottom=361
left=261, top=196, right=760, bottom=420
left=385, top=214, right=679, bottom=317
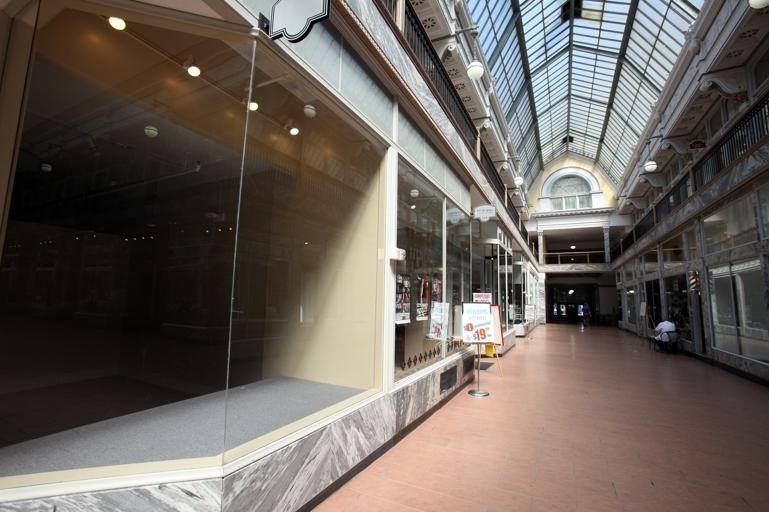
left=654, top=331, right=682, bottom=351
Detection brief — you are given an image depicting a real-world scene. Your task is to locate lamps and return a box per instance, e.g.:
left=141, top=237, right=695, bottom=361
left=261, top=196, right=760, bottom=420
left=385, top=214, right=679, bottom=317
left=644, top=135, right=662, bottom=172
left=456, top=25, right=484, bottom=79
left=508, top=155, right=523, bottom=186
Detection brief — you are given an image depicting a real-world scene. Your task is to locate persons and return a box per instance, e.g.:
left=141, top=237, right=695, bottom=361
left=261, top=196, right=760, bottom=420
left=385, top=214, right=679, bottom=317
left=582, top=303, right=592, bottom=325
left=654, top=320, right=676, bottom=352
